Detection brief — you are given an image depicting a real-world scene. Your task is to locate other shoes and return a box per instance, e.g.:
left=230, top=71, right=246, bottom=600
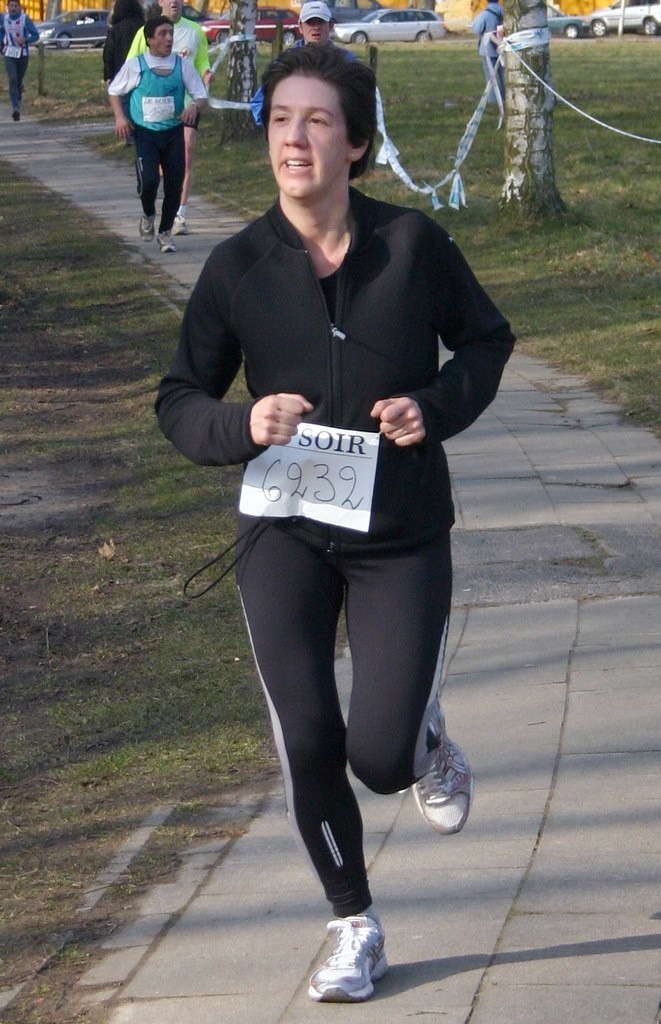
left=12, top=110, right=20, bottom=120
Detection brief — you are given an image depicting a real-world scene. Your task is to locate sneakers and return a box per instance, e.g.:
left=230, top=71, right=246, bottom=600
left=172, top=216, right=188, bottom=235
left=413, top=694, right=474, bottom=835
left=140, top=215, right=155, bottom=242
left=158, top=231, right=176, bottom=252
left=309, top=915, right=387, bottom=1002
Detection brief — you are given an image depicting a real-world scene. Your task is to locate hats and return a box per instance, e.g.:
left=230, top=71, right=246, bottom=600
left=299, top=1, right=332, bottom=21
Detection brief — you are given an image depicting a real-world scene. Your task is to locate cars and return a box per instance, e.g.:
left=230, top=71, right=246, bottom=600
left=28, top=9, right=111, bottom=50
left=182, top=5, right=216, bottom=24
left=329, top=8, right=446, bottom=45
left=588, top=0, right=661, bottom=38
left=199, top=6, right=304, bottom=46
left=546, top=2, right=588, bottom=40
left=301, top=0, right=385, bottom=26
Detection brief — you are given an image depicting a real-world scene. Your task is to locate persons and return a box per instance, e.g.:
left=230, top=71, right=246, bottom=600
left=0, top=0, right=213, bottom=252
left=249, top=2, right=359, bottom=130
left=154, top=40, right=518, bottom=1000
left=471, top=0, right=507, bottom=104
